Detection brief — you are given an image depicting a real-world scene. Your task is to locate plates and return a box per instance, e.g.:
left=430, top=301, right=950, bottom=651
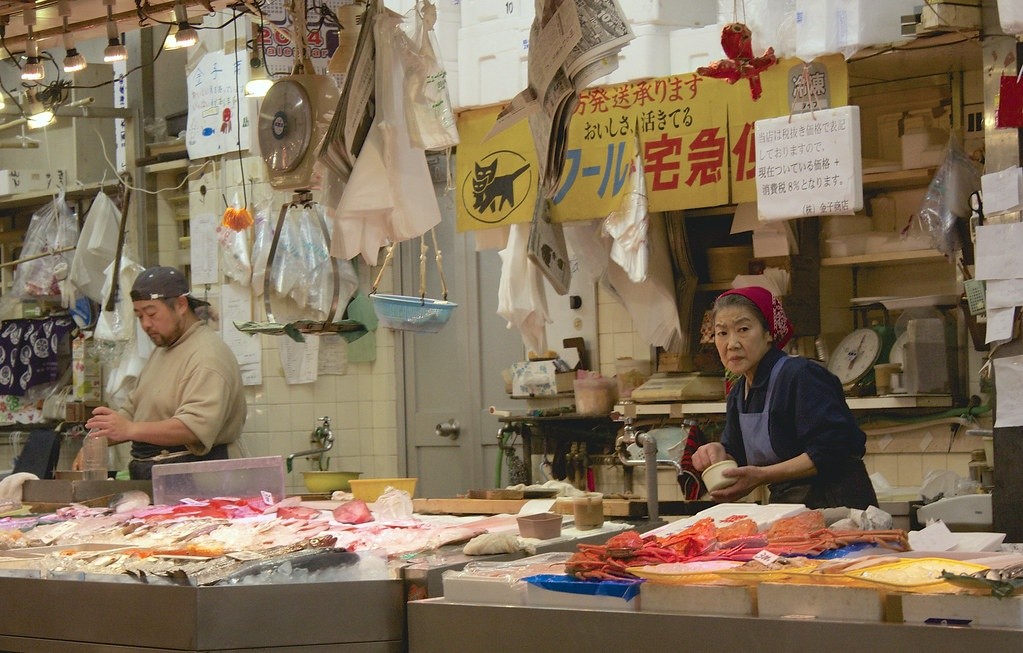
left=890, top=331, right=909, bottom=370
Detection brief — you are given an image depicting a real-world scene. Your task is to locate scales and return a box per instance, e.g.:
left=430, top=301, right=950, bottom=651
left=630, top=372, right=725, bottom=403
left=827, top=295, right=897, bottom=397
left=878, top=295, right=963, bottom=394
left=232, top=0, right=366, bottom=345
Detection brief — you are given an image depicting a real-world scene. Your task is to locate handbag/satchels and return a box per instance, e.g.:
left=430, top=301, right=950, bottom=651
left=13, top=189, right=80, bottom=302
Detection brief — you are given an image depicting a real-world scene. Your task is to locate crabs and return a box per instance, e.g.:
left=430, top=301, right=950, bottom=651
left=549, top=505, right=914, bottom=582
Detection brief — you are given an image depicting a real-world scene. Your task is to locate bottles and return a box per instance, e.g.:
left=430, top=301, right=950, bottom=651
left=82, top=427, right=108, bottom=482
left=968, top=449, right=988, bottom=481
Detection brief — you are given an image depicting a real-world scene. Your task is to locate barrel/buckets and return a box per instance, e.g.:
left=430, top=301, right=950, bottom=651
left=572, top=359, right=651, bottom=414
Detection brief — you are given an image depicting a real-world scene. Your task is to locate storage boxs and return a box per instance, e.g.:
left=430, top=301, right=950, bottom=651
left=448, top=529, right=1023, bottom=630
left=921, top=4, right=981, bottom=34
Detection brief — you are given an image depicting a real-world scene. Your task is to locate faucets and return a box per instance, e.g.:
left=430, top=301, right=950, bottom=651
left=615, top=417, right=636, bottom=451
left=497, top=422, right=522, bottom=439
left=314, top=415, right=334, bottom=441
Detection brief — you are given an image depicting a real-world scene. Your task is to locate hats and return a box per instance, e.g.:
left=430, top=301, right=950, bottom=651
left=130, top=265, right=213, bottom=308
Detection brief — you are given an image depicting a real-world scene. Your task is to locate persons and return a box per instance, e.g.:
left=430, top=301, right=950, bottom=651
left=72, top=267, right=246, bottom=482
left=691, top=286, right=879, bottom=512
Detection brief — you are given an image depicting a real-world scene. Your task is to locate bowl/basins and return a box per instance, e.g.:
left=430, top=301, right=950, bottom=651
left=347, top=477, right=419, bottom=503
left=300, top=470, right=362, bottom=496
left=700, top=460, right=738, bottom=493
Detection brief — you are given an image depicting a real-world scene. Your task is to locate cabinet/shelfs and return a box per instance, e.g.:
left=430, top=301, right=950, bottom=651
left=662, top=74, right=970, bottom=395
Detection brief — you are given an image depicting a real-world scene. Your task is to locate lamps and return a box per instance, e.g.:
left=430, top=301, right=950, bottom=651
left=244, top=22, right=274, bottom=98
left=58, top=0, right=86, bottom=72
left=174, top=0, right=198, bottom=47
left=103, top=0, right=128, bottom=62
left=163, top=8, right=181, bottom=50
left=20, top=9, right=46, bottom=82
left=24, top=87, right=57, bottom=129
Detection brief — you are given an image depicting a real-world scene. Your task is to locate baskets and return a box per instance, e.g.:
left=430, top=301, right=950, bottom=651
left=369, top=293, right=458, bottom=334
left=625, top=426, right=691, bottom=464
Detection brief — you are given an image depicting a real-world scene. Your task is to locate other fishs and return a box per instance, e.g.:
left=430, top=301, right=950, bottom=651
left=0, top=497, right=410, bottom=587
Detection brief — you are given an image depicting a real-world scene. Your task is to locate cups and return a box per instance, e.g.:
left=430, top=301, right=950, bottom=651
left=890, top=372, right=906, bottom=394
left=873, top=363, right=901, bottom=398
left=574, top=492, right=606, bottom=531
